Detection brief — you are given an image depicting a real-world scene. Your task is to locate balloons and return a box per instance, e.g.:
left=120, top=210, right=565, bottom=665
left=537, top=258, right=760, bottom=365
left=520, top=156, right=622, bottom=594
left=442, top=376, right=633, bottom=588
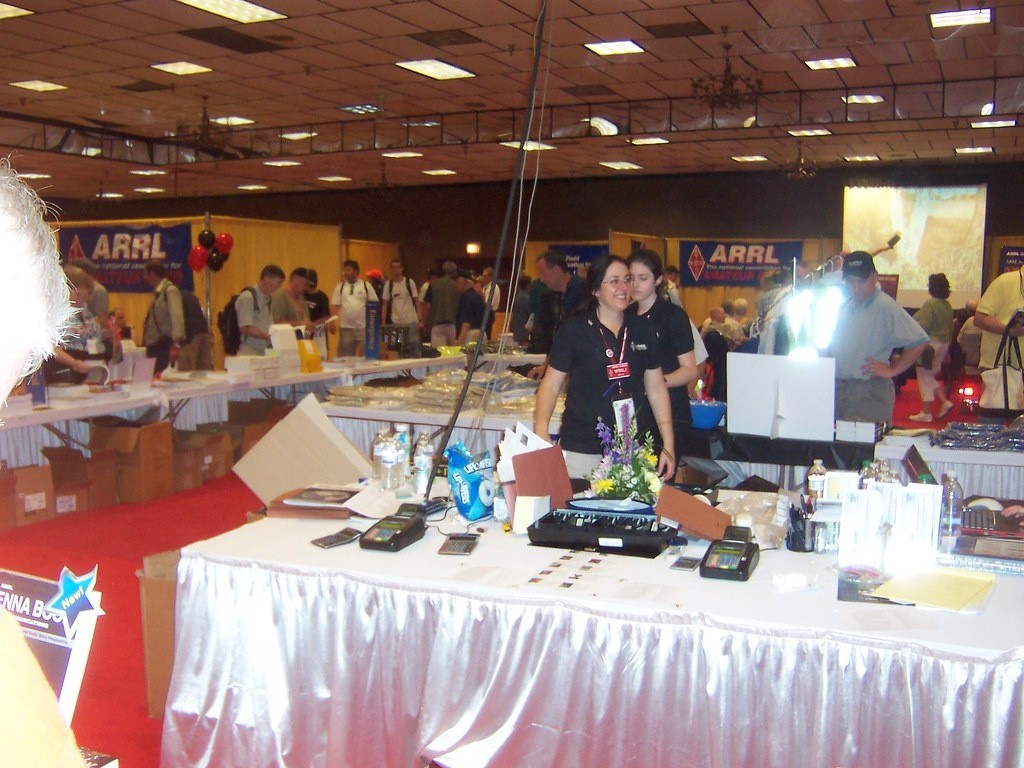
left=188, top=230, right=233, bottom=273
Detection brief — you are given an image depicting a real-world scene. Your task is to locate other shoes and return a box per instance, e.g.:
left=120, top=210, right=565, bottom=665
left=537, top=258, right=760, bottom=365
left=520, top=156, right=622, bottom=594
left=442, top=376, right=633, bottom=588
left=936, top=400, right=955, bottom=421
left=909, top=409, right=933, bottom=422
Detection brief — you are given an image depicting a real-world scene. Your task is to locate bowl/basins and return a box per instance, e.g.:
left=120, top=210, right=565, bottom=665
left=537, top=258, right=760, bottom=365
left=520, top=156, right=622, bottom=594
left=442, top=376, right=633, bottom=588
left=688, top=398, right=726, bottom=429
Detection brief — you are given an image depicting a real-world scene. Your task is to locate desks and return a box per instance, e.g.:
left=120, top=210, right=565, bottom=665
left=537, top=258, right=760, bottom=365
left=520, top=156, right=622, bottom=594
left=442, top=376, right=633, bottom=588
left=160, top=514, right=1024, bottom=768
left=0, top=380, right=162, bottom=446
left=474, top=353, right=549, bottom=370
left=683, top=429, right=874, bottom=495
left=325, top=354, right=466, bottom=387
left=874, top=443, right=1024, bottom=500
left=321, top=400, right=563, bottom=462
left=151, top=361, right=347, bottom=424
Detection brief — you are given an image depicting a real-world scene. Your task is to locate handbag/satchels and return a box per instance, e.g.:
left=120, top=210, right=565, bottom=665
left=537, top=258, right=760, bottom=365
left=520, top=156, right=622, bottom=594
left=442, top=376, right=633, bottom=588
left=979, top=310, right=1024, bottom=418
left=917, top=345, right=936, bottom=369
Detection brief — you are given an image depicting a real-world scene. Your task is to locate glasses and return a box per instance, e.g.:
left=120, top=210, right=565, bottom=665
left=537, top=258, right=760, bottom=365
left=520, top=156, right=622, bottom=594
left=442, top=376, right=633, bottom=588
left=602, top=277, right=634, bottom=288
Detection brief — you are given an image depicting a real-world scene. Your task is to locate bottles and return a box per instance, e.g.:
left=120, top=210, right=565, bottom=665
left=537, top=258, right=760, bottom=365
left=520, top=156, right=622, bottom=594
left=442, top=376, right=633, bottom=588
left=857, top=459, right=902, bottom=490
left=382, top=437, right=399, bottom=489
left=917, top=473, right=933, bottom=484
left=393, top=426, right=411, bottom=478
left=941, top=472, right=963, bottom=535
left=815, top=499, right=842, bottom=554
left=808, top=459, right=826, bottom=501
left=413, top=430, right=434, bottom=494
left=370, top=431, right=385, bottom=480
left=394, top=437, right=405, bottom=485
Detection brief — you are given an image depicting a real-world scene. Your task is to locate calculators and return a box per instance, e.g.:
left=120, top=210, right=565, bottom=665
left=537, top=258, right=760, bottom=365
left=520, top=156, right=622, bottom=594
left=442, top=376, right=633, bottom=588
left=438, top=532, right=481, bottom=555
left=310, top=527, right=362, bottom=549
left=669, top=556, right=702, bottom=571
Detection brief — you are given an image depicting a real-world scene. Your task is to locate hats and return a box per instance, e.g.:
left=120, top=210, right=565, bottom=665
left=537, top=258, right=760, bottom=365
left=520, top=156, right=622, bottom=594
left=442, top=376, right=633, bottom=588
left=451, top=270, right=477, bottom=282
left=841, top=251, right=876, bottom=281
left=365, top=269, right=385, bottom=281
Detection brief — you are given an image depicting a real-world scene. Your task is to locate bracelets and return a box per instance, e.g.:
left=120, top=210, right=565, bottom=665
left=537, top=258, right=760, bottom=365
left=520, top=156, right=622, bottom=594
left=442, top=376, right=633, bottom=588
left=173, top=342, right=181, bottom=348
left=72, top=360, right=84, bottom=371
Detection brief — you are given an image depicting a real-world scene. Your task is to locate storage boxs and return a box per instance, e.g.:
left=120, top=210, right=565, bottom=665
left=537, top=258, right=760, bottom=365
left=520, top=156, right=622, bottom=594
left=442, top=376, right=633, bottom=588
left=0, top=323, right=323, bottom=529
left=365, top=299, right=422, bottom=385
left=134, top=549, right=181, bottom=722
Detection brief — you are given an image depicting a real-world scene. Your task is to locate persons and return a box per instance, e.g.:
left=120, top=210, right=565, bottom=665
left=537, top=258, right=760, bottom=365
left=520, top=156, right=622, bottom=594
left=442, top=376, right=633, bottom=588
left=0, top=163, right=94, bottom=768
left=1002, top=505, right=1024, bottom=527
left=50, top=248, right=1024, bottom=483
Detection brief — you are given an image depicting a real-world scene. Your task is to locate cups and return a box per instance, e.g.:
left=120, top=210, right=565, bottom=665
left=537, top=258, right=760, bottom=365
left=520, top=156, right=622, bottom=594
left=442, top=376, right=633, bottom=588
left=786, top=518, right=814, bottom=551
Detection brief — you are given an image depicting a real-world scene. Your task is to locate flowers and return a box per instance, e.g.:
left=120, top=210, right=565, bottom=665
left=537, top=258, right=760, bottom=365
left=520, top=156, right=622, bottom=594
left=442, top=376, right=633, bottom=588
left=586, top=402, right=665, bottom=505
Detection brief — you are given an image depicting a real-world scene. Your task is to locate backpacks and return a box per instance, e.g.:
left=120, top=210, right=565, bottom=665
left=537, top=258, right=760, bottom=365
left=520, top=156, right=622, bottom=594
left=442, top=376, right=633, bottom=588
left=216, top=285, right=272, bottom=356
left=165, top=284, right=194, bottom=344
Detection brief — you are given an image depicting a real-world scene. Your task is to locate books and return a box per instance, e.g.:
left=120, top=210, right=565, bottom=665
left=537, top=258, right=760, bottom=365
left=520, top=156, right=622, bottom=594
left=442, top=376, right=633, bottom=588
left=0, top=570, right=119, bottom=768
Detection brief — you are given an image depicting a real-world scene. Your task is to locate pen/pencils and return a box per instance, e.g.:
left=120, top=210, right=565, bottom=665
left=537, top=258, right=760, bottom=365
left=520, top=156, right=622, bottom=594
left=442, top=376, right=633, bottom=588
left=787, top=494, right=815, bottom=551
